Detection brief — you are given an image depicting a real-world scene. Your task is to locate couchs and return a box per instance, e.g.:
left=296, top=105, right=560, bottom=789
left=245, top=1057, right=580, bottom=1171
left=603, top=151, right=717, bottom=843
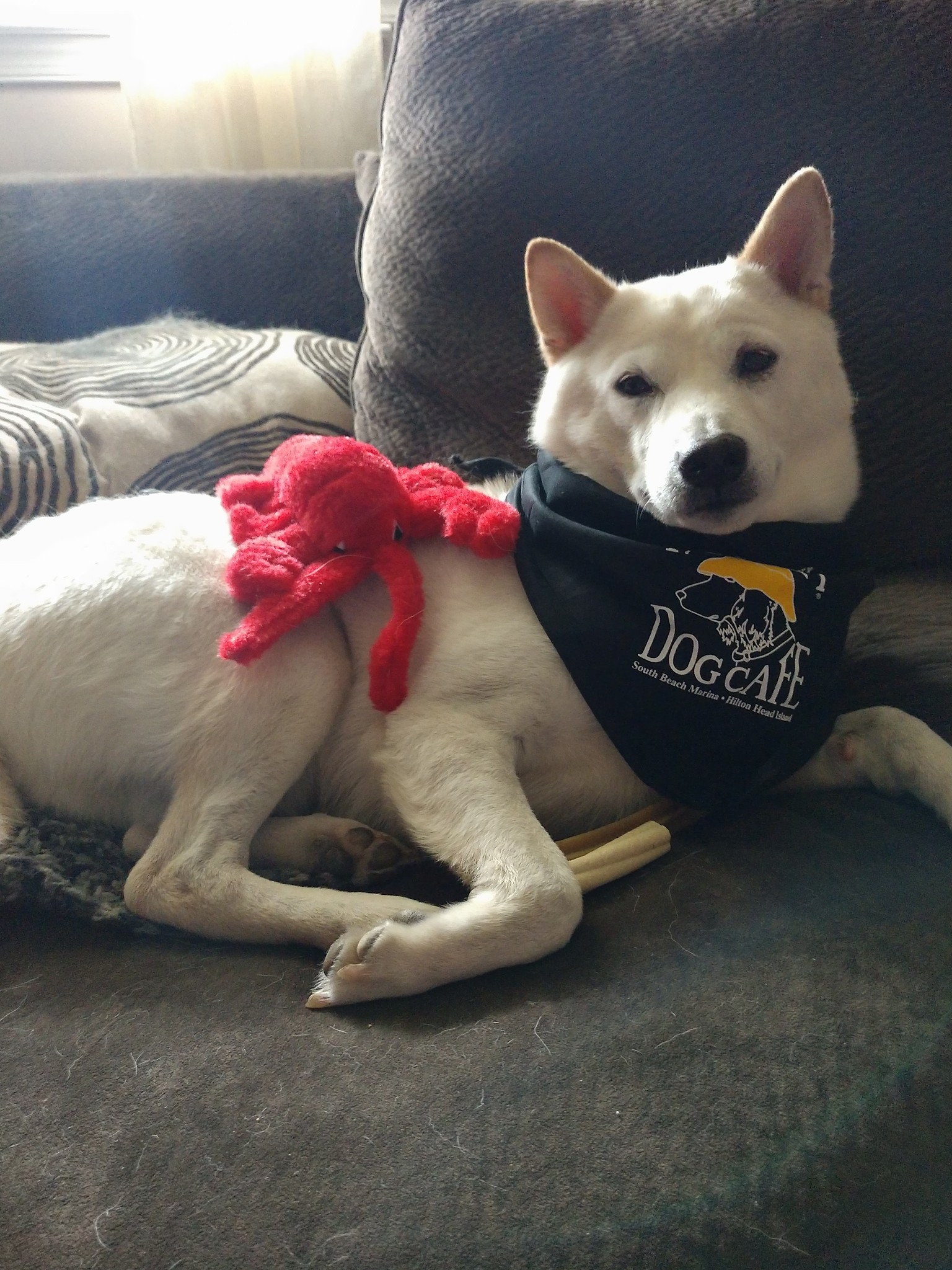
left=1, top=174, right=952, bottom=1270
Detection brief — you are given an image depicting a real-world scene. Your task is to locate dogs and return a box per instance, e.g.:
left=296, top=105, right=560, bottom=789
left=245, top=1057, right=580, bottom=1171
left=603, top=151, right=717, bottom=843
left=1, top=163, right=952, bottom=1016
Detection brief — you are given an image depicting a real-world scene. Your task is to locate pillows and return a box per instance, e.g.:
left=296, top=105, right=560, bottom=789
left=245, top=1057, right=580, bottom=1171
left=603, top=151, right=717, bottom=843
left=350, top=1, right=952, bottom=499
